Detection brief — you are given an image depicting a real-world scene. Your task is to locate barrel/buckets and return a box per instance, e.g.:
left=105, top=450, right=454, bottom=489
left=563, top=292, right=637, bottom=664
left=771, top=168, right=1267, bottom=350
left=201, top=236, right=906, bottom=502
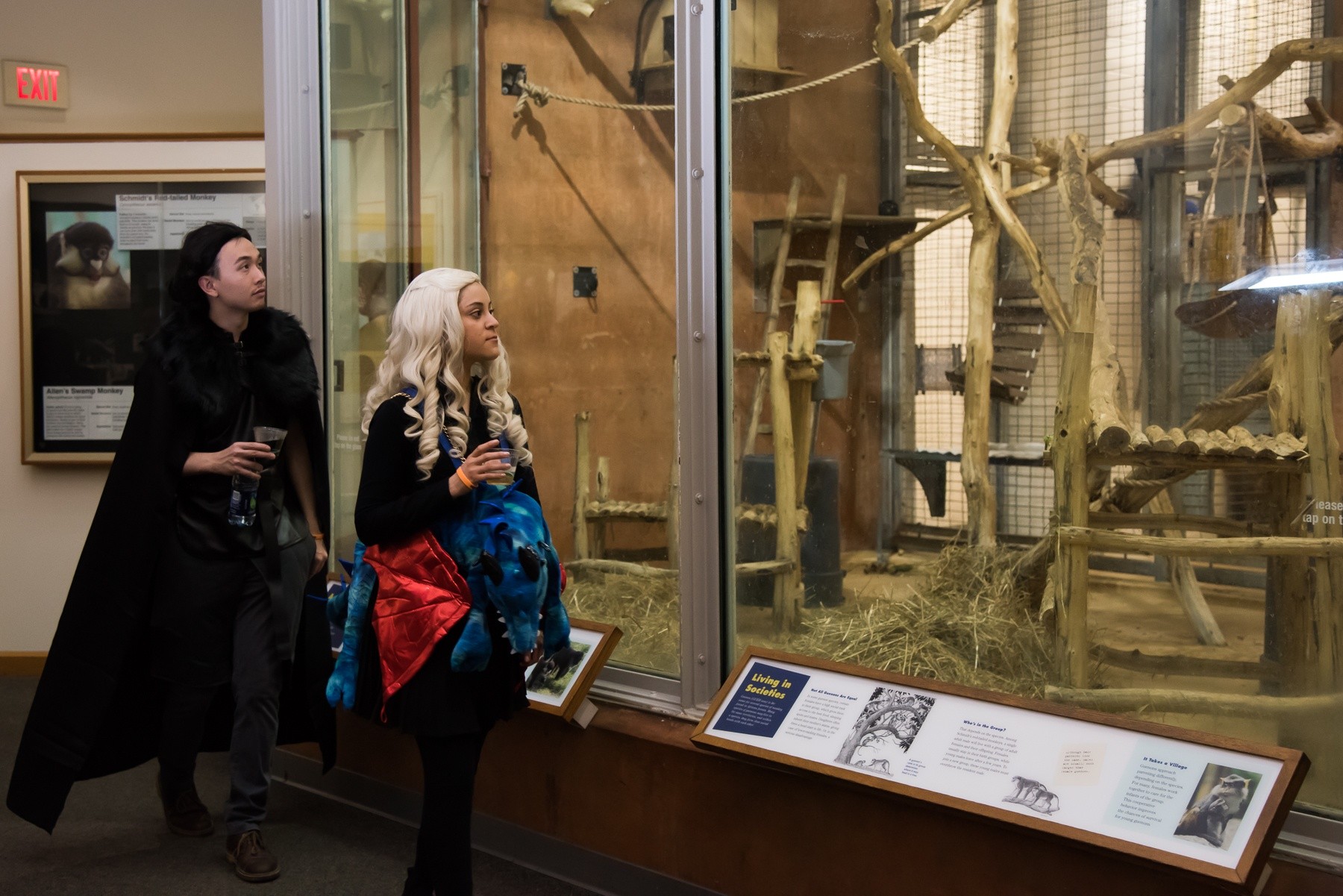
left=811, top=340, right=855, bottom=401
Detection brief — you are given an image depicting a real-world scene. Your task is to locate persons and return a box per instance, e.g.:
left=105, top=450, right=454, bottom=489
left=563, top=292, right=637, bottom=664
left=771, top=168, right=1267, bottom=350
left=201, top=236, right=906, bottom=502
left=326, top=268, right=570, bottom=896
left=6, top=224, right=336, bottom=879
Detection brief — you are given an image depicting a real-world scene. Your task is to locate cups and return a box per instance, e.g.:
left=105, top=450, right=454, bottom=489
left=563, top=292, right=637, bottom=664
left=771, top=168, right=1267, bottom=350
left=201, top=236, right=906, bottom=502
left=252, top=426, right=288, bottom=458
left=483, top=448, right=520, bottom=485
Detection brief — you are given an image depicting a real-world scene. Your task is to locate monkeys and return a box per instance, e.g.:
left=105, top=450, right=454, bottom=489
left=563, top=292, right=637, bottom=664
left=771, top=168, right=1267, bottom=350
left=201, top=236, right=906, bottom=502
left=1174, top=774, right=1251, bottom=848
left=1011, top=776, right=1060, bottom=815
left=526, top=647, right=583, bottom=690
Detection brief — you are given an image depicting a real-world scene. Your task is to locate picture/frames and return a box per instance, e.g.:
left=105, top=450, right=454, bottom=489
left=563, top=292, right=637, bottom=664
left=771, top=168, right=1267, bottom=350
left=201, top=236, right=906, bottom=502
left=524, top=617, right=625, bottom=721
left=16, top=168, right=265, bottom=462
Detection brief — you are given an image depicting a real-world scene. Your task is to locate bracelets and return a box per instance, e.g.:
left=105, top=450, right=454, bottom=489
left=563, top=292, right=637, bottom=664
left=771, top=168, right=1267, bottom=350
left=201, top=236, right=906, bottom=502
left=455, top=466, right=478, bottom=489
left=312, top=532, right=323, bottom=539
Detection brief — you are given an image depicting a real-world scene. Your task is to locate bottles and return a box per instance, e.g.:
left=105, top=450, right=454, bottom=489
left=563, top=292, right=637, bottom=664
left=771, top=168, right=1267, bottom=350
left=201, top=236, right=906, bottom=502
left=228, top=458, right=259, bottom=526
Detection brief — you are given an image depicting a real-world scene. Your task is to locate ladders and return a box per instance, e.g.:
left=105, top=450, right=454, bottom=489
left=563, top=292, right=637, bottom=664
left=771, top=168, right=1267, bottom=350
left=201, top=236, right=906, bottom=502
left=742, top=171, right=850, bottom=452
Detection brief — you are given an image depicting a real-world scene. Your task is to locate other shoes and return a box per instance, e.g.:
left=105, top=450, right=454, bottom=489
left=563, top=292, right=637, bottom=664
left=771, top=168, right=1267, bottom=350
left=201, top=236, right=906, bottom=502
left=225, top=831, right=282, bottom=882
left=156, top=774, right=214, bottom=837
